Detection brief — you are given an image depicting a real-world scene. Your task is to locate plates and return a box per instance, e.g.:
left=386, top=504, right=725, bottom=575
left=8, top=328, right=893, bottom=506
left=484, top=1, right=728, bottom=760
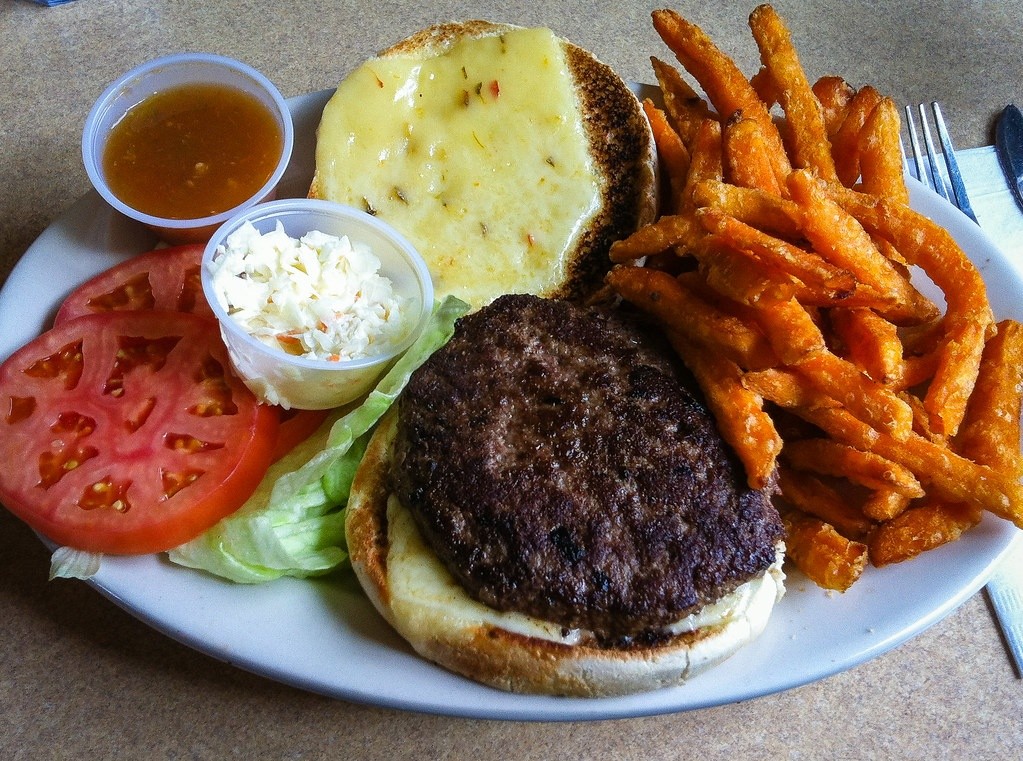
left=0, top=85, right=1016, bottom=720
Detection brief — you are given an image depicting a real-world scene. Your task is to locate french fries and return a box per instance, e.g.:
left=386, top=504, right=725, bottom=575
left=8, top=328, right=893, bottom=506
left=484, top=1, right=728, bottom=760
left=608, top=1, right=1023, bottom=593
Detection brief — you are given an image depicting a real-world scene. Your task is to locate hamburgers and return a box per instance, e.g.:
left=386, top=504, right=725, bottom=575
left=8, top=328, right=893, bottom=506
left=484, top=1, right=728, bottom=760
left=309, top=19, right=785, bottom=697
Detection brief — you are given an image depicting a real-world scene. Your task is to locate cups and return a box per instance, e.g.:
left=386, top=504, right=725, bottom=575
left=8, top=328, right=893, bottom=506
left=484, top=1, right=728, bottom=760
left=80, top=52, right=294, bottom=242
left=197, top=197, right=433, bottom=411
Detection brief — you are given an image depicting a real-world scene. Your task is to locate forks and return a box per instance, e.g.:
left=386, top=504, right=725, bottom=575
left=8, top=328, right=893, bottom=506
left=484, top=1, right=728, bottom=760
left=894, top=98, right=1022, bottom=674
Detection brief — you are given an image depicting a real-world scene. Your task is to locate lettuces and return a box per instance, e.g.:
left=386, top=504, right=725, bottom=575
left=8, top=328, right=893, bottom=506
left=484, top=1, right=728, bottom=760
left=168, top=294, right=468, bottom=583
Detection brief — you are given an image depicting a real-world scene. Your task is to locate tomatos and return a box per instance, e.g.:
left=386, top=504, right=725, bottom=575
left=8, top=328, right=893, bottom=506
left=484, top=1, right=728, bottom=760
left=0, top=244, right=322, bottom=555
